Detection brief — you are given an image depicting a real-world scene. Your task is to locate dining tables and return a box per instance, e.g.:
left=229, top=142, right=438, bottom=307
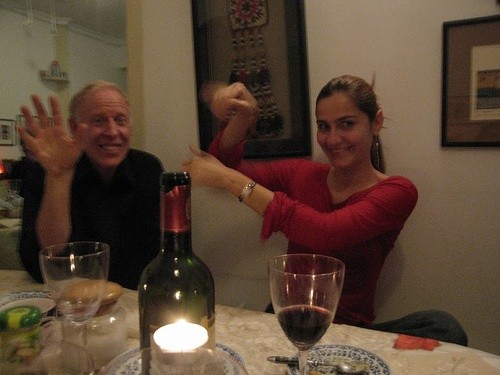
left=0, top=269, right=500, bottom=375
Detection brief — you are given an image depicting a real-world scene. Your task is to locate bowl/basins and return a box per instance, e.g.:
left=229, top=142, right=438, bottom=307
left=68, top=280, right=123, bottom=317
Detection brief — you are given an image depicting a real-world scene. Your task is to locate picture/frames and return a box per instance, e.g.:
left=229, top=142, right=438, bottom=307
left=440, top=14, right=500, bottom=147
left=0, top=115, right=53, bottom=146
left=191, top=0, right=312, bottom=167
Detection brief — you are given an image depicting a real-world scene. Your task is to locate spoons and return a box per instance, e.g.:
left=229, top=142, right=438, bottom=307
left=267, top=356, right=367, bottom=373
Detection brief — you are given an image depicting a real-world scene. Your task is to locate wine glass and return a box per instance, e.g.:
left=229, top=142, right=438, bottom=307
left=39, top=242, right=110, bottom=348
left=269, top=254, right=345, bottom=375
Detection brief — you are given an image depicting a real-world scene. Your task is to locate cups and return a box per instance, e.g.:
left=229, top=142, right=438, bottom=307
left=63, top=308, right=127, bottom=371
left=34, top=342, right=96, bottom=374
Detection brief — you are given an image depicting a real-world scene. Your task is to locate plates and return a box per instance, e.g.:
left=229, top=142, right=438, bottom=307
left=287, top=344, right=391, bottom=375
left=0, top=292, right=56, bottom=319
left=104, top=342, right=248, bottom=375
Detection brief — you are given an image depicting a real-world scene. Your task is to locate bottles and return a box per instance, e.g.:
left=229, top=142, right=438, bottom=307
left=139, top=171, right=215, bottom=375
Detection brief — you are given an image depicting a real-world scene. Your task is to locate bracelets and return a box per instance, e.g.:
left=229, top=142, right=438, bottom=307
left=238, top=181, right=256, bottom=203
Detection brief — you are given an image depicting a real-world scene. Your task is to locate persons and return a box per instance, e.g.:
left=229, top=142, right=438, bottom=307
left=8, top=80, right=166, bottom=291
left=181, top=75, right=418, bottom=329
